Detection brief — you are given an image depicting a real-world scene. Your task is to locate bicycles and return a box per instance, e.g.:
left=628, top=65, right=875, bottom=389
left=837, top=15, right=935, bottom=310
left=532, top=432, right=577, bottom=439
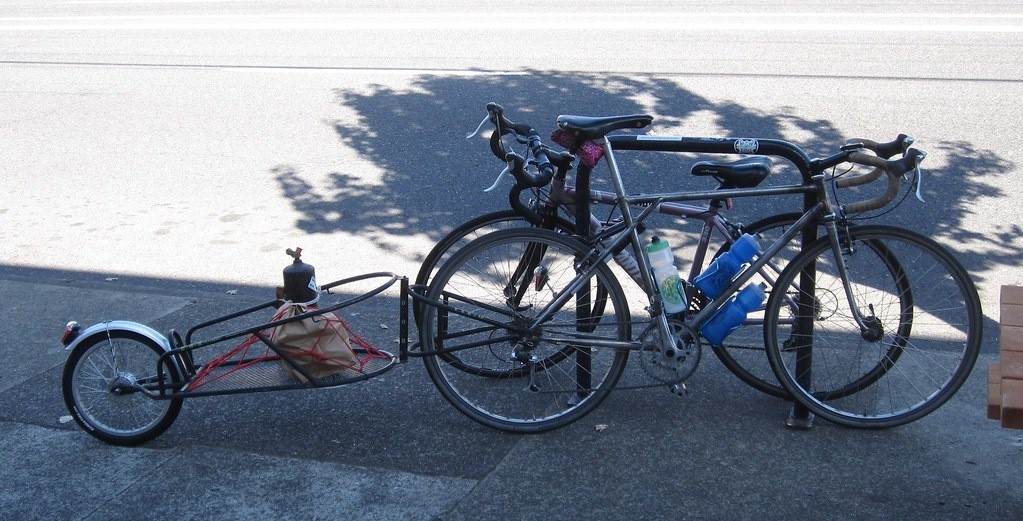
left=414, top=103, right=983, bottom=432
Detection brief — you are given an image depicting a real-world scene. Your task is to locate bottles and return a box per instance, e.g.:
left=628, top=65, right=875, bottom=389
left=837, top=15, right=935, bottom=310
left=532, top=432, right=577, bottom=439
left=700, top=281, right=769, bottom=347
left=646, top=236, right=687, bottom=314
left=693, top=232, right=762, bottom=298
